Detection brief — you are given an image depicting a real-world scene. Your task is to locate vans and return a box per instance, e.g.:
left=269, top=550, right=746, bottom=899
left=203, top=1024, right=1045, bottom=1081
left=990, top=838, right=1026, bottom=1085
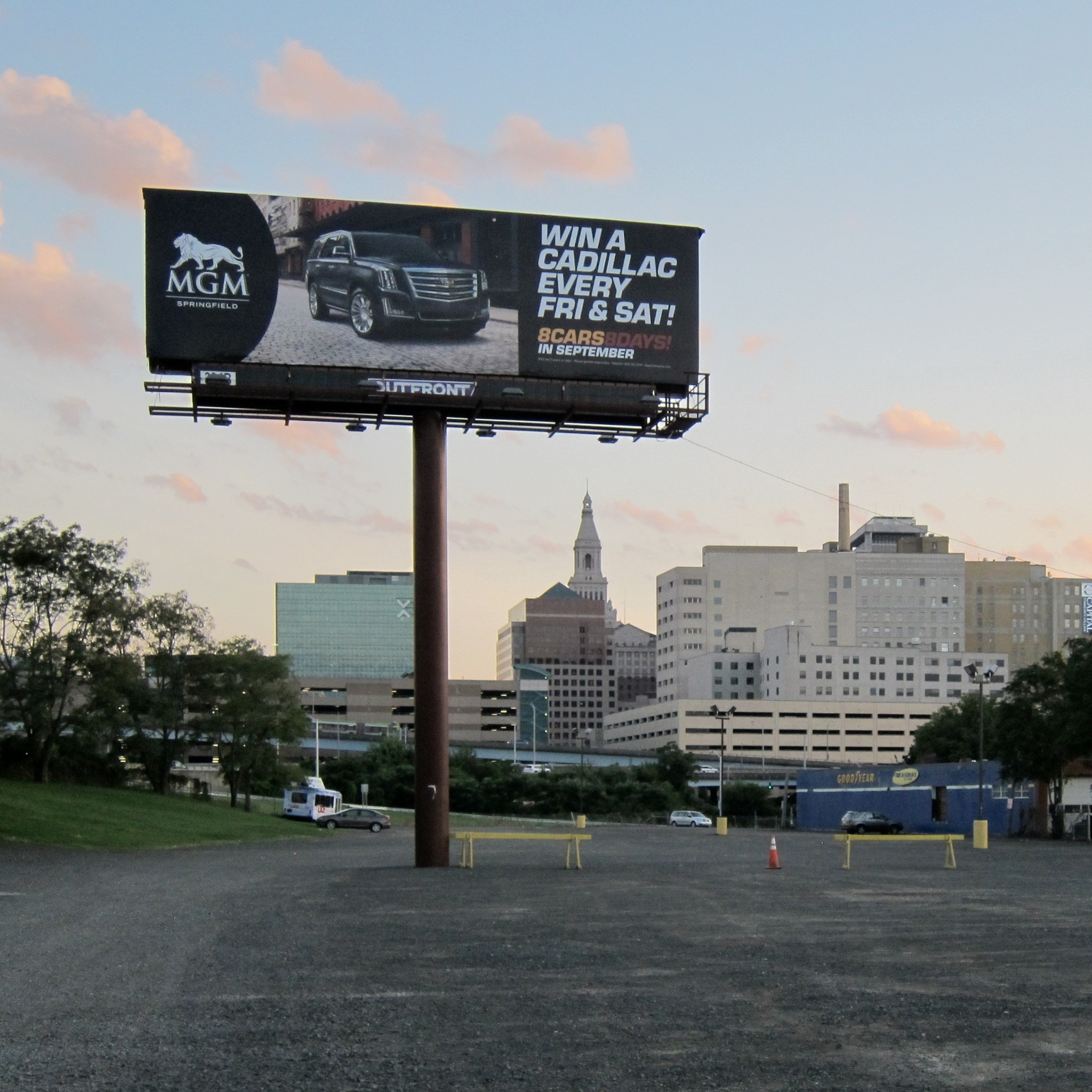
left=669, top=810, right=712, bottom=828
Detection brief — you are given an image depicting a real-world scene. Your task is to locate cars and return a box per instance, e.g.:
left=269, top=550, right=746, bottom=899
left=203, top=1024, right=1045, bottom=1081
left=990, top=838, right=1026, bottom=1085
left=694, top=765, right=718, bottom=773
left=524, top=764, right=551, bottom=774
left=316, top=808, right=391, bottom=833
left=840, top=811, right=904, bottom=834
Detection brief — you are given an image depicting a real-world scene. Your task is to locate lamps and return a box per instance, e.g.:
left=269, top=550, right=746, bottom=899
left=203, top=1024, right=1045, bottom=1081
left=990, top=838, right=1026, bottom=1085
left=205, top=373, right=660, bottom=443
left=572, top=728, right=592, bottom=736
left=710, top=705, right=736, bottom=719
left=964, top=663, right=998, bottom=683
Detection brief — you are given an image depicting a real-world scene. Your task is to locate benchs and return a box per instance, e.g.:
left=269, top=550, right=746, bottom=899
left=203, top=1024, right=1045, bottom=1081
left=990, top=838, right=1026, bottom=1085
left=834, top=834, right=964, bottom=869
left=452, top=831, right=591, bottom=870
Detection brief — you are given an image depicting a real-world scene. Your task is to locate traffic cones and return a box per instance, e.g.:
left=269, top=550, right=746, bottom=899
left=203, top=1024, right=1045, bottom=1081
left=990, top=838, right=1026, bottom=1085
left=766, top=836, right=782, bottom=869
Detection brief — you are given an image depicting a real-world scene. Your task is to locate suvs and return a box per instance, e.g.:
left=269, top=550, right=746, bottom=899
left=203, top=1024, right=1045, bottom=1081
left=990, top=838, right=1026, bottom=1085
left=304, top=230, right=491, bottom=341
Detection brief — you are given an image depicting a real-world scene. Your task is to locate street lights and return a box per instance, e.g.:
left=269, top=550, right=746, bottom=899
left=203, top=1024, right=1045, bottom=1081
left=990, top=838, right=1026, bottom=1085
left=571, top=727, right=592, bottom=815
left=710, top=704, right=737, bottom=817
left=964, top=662, right=999, bottom=819
